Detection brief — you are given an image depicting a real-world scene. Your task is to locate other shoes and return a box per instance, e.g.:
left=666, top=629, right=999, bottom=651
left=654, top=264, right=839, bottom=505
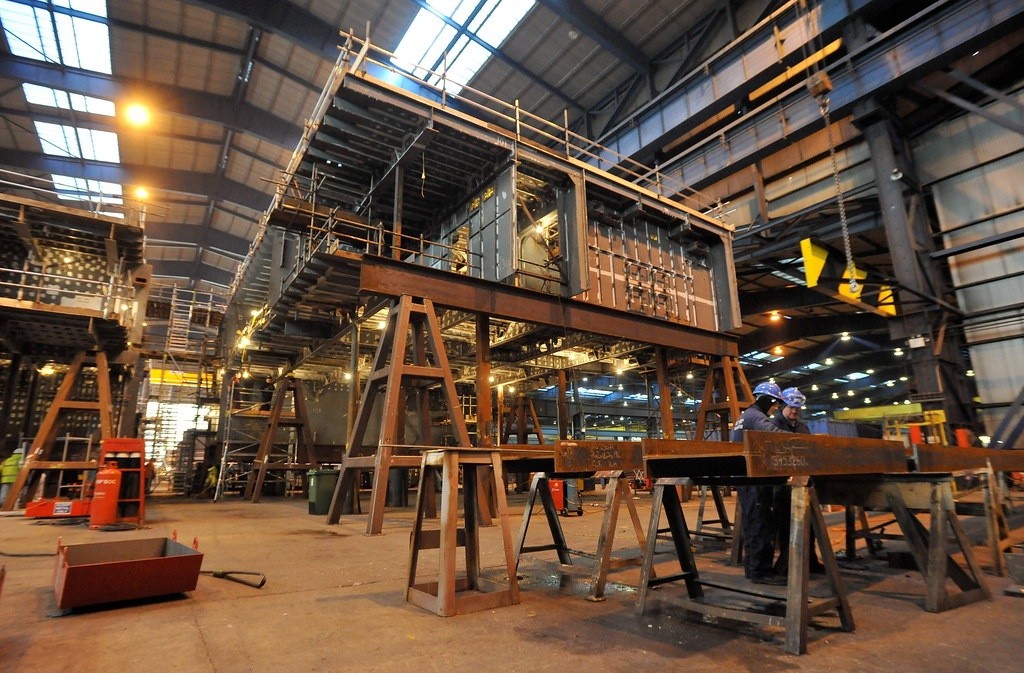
left=773, top=554, right=828, bottom=574
left=747, top=569, right=788, bottom=586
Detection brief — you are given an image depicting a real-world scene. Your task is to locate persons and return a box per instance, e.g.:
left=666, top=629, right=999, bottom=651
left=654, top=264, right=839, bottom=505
left=185, top=463, right=209, bottom=499
left=260, top=374, right=276, bottom=412
left=767, top=386, right=817, bottom=575
left=145, top=457, right=157, bottom=495
left=731, top=383, right=795, bottom=582
left=0, top=449, right=24, bottom=507
left=229, top=372, right=242, bottom=409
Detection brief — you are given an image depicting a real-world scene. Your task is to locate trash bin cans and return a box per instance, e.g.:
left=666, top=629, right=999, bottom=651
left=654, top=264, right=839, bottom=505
left=305, top=468, right=354, bottom=515
left=548, top=478, right=583, bottom=517
left=385, top=466, right=409, bottom=507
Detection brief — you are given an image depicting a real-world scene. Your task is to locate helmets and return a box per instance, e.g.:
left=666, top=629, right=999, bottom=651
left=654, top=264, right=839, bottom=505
left=780, top=387, right=806, bottom=407
left=752, top=382, right=784, bottom=400
